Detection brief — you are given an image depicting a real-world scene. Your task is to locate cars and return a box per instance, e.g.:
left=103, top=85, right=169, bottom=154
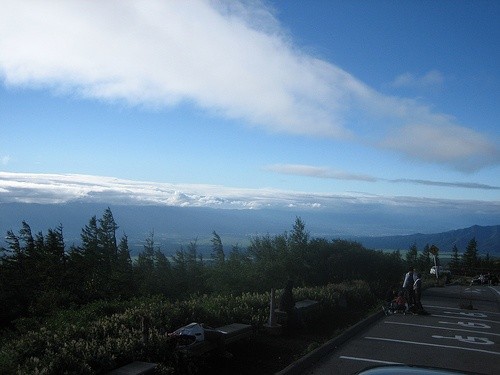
left=430, top=266, right=453, bottom=280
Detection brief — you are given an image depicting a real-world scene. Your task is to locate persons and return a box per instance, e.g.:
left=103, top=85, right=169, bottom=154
left=384, top=266, right=425, bottom=316
left=480, top=272, right=486, bottom=285
left=486, top=272, right=492, bottom=286
